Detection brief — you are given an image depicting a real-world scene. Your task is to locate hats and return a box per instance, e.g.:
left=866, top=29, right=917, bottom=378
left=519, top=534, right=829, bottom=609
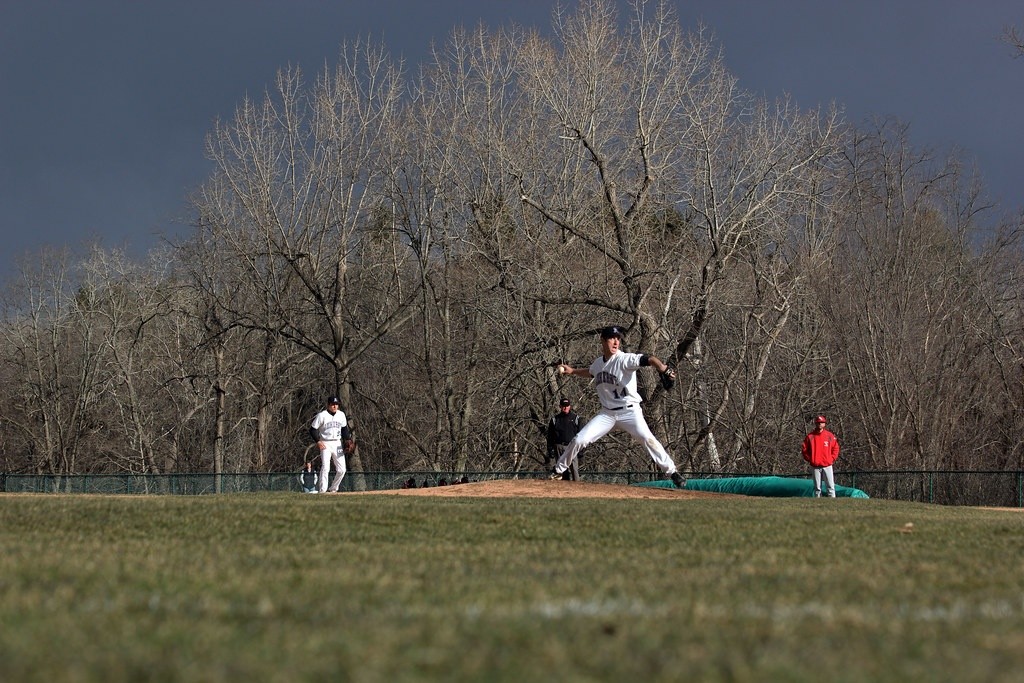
left=328, top=396, right=340, bottom=404
left=560, top=398, right=570, bottom=406
left=602, top=327, right=624, bottom=339
left=815, top=416, right=826, bottom=422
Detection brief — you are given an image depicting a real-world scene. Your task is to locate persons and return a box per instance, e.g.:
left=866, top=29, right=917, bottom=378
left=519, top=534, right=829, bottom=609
left=547, top=398, right=582, bottom=481
left=310, top=396, right=355, bottom=493
left=802, top=416, right=840, bottom=498
left=300, top=461, right=318, bottom=493
left=547, top=326, right=687, bottom=487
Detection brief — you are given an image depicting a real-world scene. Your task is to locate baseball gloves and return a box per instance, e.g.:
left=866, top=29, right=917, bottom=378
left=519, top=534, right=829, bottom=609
left=659, top=365, right=675, bottom=390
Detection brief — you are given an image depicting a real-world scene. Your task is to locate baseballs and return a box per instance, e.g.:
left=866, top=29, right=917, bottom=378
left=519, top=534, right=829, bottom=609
left=558, top=367, right=565, bottom=374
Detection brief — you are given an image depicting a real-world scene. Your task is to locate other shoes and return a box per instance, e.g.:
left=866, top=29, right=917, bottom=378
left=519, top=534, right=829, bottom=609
left=539, top=469, right=563, bottom=480
left=671, top=472, right=687, bottom=487
